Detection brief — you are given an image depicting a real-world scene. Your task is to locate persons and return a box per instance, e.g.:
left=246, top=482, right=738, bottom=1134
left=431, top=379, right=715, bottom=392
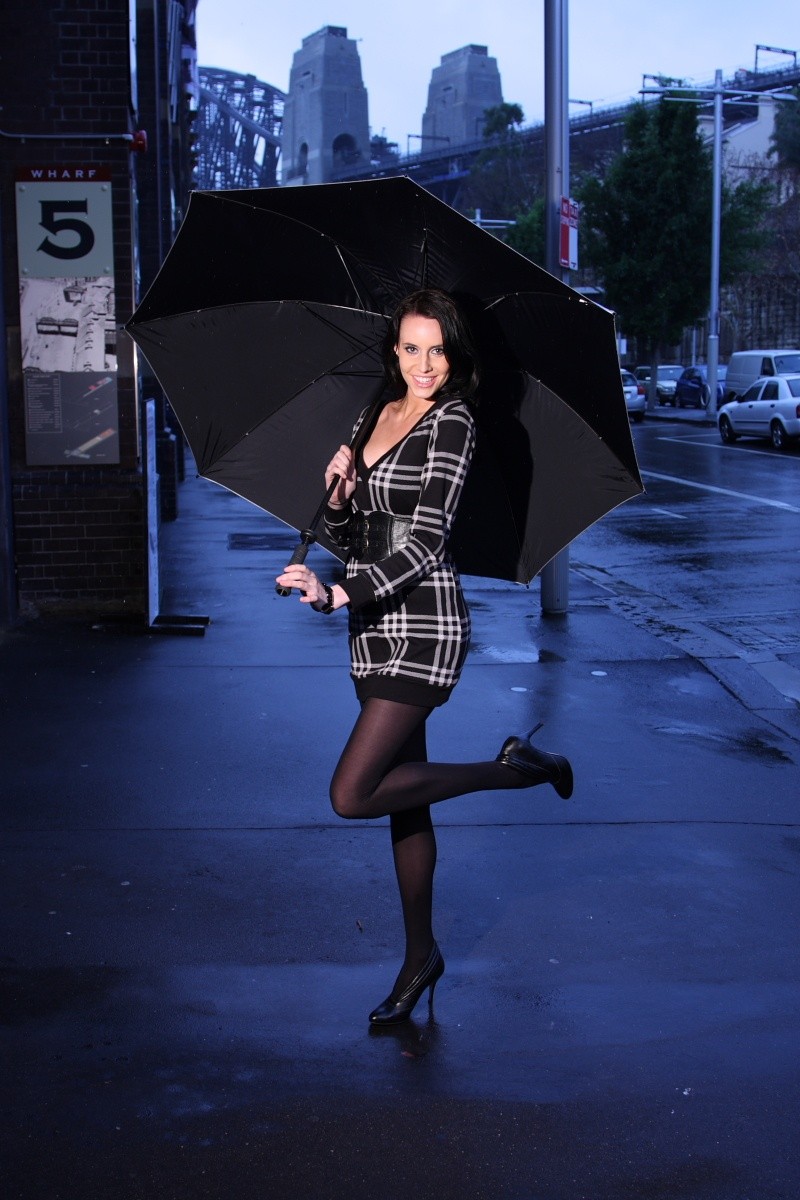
left=274, top=292, right=573, bottom=1025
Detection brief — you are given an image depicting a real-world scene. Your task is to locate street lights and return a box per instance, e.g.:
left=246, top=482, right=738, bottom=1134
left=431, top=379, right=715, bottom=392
left=638, top=86, right=799, bottom=419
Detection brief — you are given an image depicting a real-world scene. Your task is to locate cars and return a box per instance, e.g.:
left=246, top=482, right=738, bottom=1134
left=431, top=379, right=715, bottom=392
left=673, top=365, right=728, bottom=411
left=716, top=375, right=800, bottom=449
left=621, top=368, right=646, bottom=422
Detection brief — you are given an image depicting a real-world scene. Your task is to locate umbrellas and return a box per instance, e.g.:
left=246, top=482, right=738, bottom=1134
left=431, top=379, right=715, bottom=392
left=119, top=173, right=648, bottom=597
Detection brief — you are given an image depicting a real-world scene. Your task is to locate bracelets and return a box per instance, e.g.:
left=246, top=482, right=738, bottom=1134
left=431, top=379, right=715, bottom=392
left=310, top=583, right=334, bottom=615
left=328, top=501, right=348, bottom=505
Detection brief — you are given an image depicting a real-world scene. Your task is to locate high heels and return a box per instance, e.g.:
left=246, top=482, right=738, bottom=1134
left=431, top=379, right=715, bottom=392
left=368, top=942, right=444, bottom=1023
left=495, top=723, right=573, bottom=798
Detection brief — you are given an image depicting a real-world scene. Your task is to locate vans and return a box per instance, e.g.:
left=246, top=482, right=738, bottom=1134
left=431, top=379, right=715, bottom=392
left=632, top=364, right=684, bottom=407
left=724, top=350, right=800, bottom=402
